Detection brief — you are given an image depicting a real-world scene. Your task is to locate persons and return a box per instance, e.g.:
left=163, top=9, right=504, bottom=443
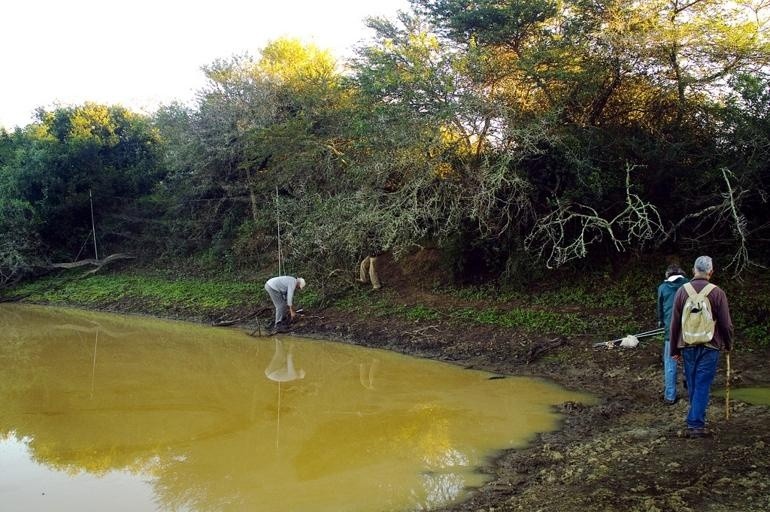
left=262, top=339, right=307, bottom=384
left=355, top=224, right=383, bottom=290
left=263, top=275, right=307, bottom=327
left=668, top=255, right=737, bottom=439
left=656, top=260, right=690, bottom=404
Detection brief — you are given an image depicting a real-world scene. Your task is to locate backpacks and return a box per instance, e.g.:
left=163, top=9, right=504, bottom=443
left=681, top=282, right=717, bottom=344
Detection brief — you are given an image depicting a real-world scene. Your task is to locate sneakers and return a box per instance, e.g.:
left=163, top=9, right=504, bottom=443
left=357, top=279, right=381, bottom=291
left=659, top=395, right=674, bottom=404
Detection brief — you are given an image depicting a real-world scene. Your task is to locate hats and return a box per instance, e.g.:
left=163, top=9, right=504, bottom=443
left=299, top=278, right=305, bottom=289
